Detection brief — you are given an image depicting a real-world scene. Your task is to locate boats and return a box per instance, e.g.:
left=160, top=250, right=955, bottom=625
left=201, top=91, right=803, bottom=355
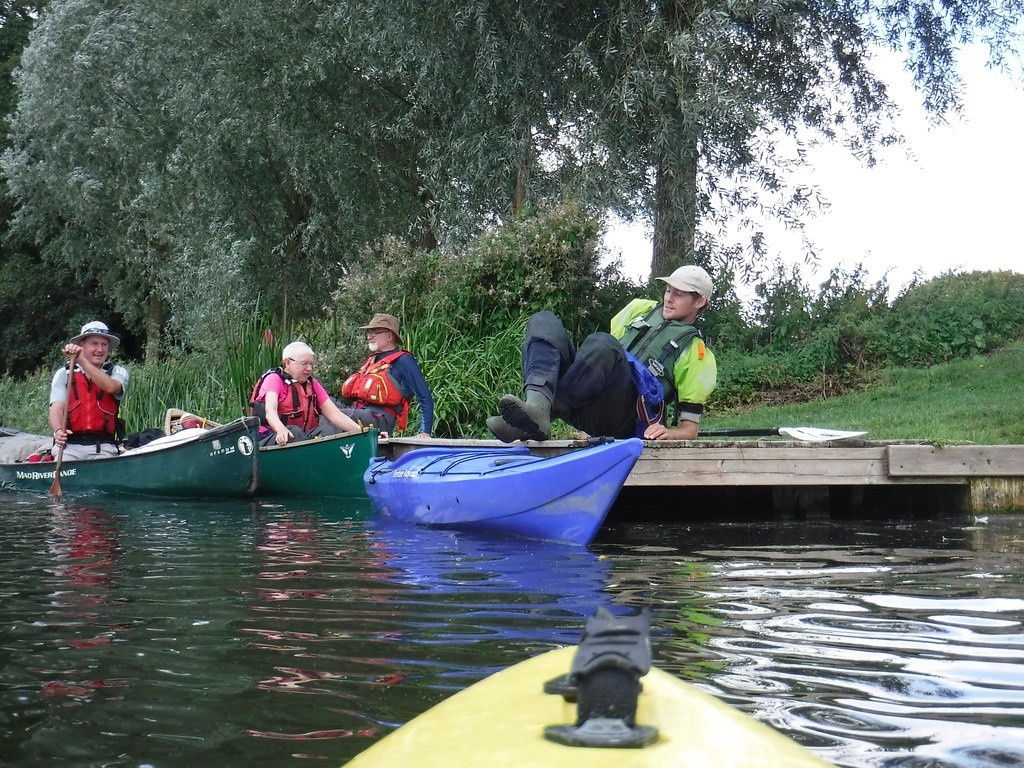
left=165, top=404, right=381, bottom=496
left=364, top=436, right=645, bottom=544
left=0, top=413, right=266, bottom=498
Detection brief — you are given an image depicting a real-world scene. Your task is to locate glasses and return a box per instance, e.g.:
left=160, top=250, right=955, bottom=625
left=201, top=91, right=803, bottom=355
left=289, top=358, right=315, bottom=368
left=83, top=328, right=110, bottom=334
left=367, top=329, right=389, bottom=334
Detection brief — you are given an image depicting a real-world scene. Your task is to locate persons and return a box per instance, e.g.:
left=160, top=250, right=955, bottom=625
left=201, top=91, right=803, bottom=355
left=486, top=266, right=717, bottom=443
left=49, top=321, right=129, bottom=462
left=249, top=314, right=433, bottom=447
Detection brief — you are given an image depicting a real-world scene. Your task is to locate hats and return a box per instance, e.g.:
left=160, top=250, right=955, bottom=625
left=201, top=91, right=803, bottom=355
left=655, top=265, right=713, bottom=303
left=70, top=321, right=120, bottom=347
left=359, top=313, right=403, bottom=346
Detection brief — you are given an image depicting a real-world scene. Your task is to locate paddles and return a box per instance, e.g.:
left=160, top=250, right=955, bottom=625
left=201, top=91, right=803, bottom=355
left=700, top=426, right=872, bottom=442
left=46, top=346, right=84, bottom=496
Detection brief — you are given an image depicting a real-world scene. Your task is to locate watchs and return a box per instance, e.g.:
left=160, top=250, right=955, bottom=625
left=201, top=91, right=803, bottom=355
left=54, top=428, right=62, bottom=439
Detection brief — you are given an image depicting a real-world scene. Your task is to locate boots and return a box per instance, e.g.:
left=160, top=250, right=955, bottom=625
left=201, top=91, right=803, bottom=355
left=498, top=389, right=551, bottom=441
left=485, top=416, right=534, bottom=443
